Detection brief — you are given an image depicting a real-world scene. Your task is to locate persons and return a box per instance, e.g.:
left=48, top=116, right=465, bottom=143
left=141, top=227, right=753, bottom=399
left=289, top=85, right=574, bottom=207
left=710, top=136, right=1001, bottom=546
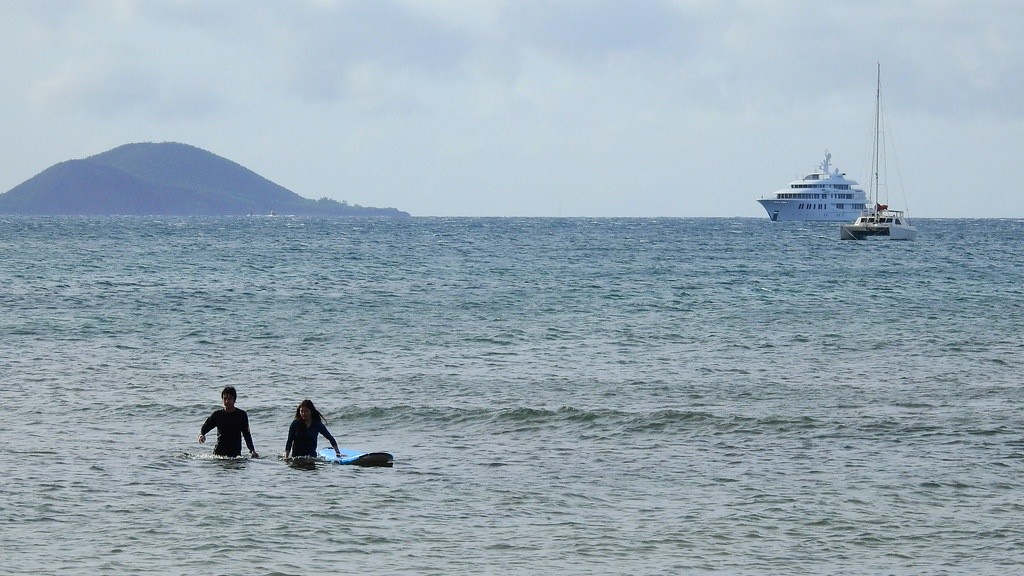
left=284, top=399, right=346, bottom=458
left=198, top=387, right=256, bottom=457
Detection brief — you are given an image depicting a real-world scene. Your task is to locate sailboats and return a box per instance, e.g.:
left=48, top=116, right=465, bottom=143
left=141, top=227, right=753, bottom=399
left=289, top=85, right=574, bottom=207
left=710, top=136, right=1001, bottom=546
left=839, top=63, right=918, bottom=240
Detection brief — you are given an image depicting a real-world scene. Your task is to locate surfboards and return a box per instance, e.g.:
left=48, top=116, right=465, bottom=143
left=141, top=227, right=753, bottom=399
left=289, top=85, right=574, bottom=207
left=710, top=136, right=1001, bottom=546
left=318, top=447, right=394, bottom=464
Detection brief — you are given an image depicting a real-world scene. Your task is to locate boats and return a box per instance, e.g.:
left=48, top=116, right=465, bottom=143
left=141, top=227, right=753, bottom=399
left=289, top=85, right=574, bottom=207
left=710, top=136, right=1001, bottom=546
left=757, top=150, right=877, bottom=221
